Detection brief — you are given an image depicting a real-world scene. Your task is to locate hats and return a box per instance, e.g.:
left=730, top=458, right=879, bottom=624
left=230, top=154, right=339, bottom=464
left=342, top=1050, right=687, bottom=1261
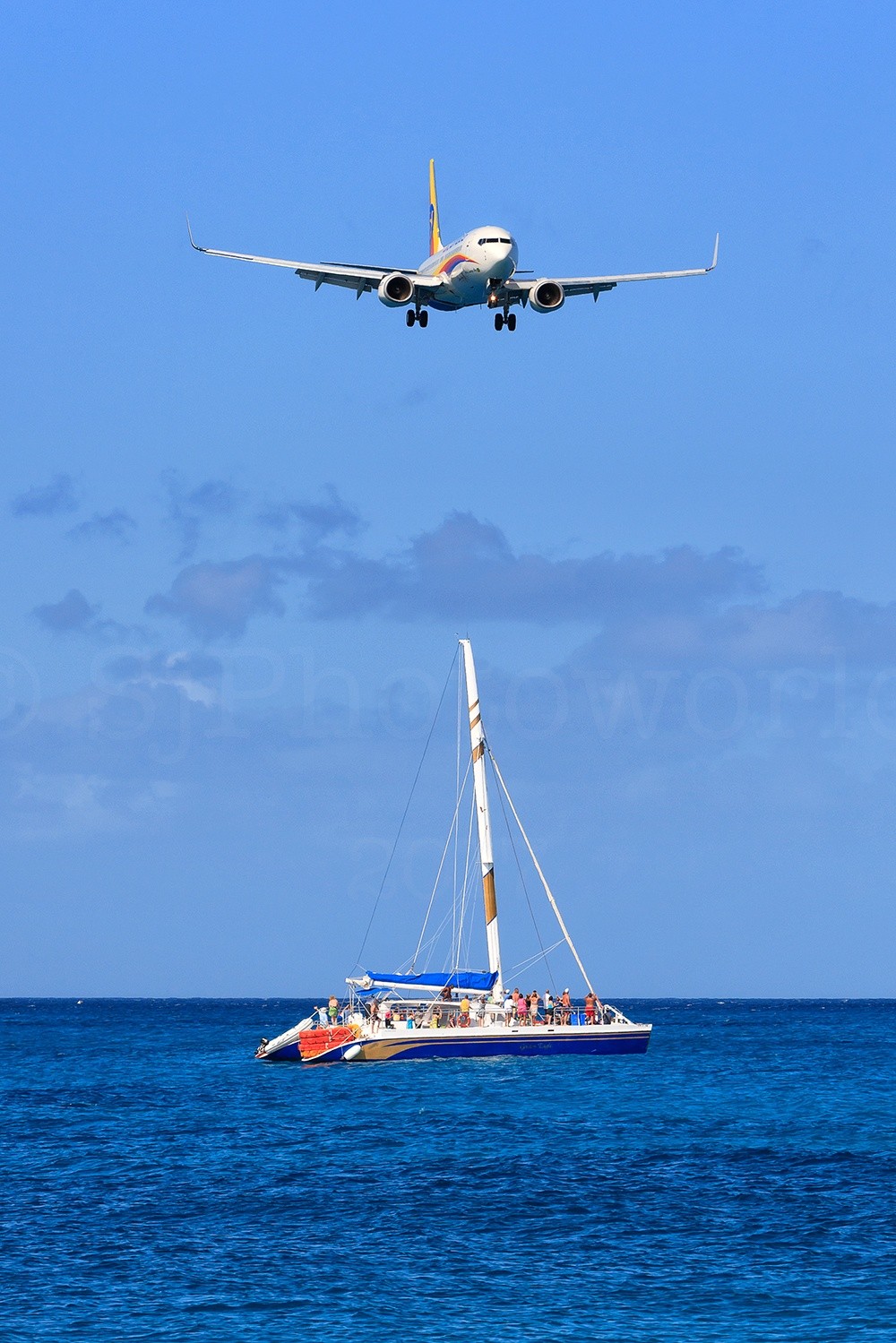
left=565, top=988, right=569, bottom=993
left=320, top=1007, right=327, bottom=1013
left=505, top=989, right=510, bottom=993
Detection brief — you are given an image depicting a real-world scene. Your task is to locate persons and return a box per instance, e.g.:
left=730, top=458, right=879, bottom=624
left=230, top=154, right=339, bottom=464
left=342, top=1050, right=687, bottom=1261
left=313, top=984, right=612, bottom=1033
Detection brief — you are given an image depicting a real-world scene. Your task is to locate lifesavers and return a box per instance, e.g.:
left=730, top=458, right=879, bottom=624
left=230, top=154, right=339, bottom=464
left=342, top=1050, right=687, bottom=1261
left=347, top=1023, right=361, bottom=1038
left=457, top=1013, right=471, bottom=1028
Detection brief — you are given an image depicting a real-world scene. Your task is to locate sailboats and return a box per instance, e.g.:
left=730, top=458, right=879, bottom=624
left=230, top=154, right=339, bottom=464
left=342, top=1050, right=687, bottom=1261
left=250, top=629, right=655, bottom=1065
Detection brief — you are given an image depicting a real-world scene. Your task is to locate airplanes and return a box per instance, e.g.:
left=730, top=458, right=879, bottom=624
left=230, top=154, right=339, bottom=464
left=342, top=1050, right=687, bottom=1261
left=186, top=159, right=722, bottom=333
